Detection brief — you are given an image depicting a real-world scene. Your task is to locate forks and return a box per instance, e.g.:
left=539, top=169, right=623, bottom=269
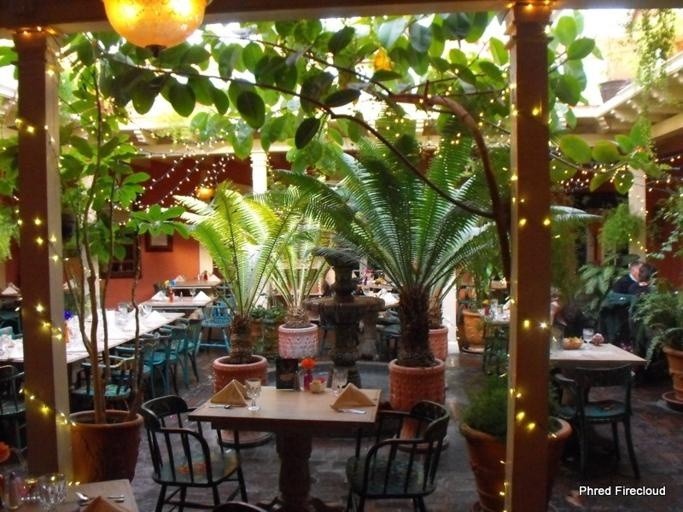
left=332, top=406, right=366, bottom=415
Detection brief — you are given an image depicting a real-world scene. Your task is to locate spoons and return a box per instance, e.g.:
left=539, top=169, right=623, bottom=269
left=73, top=489, right=125, bottom=504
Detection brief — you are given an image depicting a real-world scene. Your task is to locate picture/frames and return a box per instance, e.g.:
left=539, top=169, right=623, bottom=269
left=142, top=212, right=173, bottom=254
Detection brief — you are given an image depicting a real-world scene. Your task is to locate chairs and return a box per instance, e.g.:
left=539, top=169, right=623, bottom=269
left=1, top=268, right=682, bottom=511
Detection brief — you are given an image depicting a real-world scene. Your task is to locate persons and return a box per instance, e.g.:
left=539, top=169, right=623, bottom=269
left=549, top=261, right=657, bottom=386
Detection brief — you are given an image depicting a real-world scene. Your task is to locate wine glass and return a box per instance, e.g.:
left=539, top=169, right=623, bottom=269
left=112, top=302, right=153, bottom=328
left=332, top=367, right=348, bottom=396
left=244, top=378, right=261, bottom=411
left=582, top=328, right=594, bottom=352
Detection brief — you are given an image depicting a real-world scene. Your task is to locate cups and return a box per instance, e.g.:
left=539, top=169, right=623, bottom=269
left=0, top=470, right=67, bottom=512
left=483, top=298, right=504, bottom=318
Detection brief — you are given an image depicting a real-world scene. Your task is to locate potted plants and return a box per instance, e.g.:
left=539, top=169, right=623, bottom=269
left=243, top=120, right=606, bottom=450
left=451, top=318, right=573, bottom=512
left=241, top=184, right=351, bottom=365
left=0, top=40, right=149, bottom=481
left=155, top=188, right=308, bottom=448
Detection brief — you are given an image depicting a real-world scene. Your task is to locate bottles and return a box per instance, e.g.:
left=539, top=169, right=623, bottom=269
left=298, top=370, right=328, bottom=393
left=169, top=288, right=175, bottom=303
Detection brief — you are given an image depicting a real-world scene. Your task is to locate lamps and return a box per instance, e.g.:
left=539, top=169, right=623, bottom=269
left=100, top=0, right=209, bottom=58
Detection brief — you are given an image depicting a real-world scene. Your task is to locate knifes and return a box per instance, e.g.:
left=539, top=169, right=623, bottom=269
left=209, top=404, right=244, bottom=410
